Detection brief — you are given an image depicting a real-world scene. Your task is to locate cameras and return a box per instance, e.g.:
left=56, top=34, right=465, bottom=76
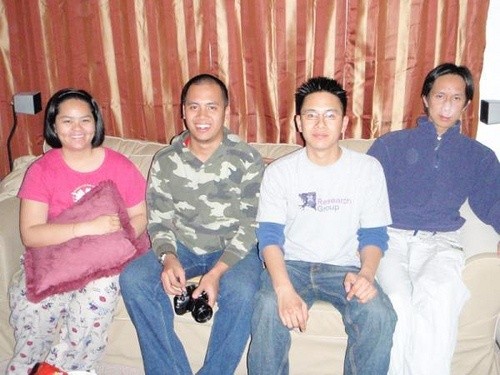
left=174, top=285, right=213, bottom=323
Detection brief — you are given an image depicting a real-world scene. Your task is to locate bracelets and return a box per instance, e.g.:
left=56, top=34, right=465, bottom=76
left=72, top=223, right=78, bottom=238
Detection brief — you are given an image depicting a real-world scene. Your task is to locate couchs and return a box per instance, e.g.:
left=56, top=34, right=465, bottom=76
left=0, top=135, right=500, bottom=375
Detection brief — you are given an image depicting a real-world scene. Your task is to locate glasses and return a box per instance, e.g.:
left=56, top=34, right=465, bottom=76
left=299, top=112, right=342, bottom=120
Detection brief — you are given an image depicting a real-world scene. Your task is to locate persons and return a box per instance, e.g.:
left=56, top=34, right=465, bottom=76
left=362, top=63, right=500, bottom=375
left=119, top=73, right=266, bottom=375
left=247, top=76, right=399, bottom=375
left=5, top=86, right=147, bottom=375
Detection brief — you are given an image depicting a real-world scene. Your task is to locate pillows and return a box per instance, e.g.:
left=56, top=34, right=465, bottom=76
left=19, top=180, right=144, bottom=304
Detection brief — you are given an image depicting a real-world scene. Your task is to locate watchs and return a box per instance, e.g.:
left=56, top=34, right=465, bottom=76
left=160, top=251, right=176, bottom=265
